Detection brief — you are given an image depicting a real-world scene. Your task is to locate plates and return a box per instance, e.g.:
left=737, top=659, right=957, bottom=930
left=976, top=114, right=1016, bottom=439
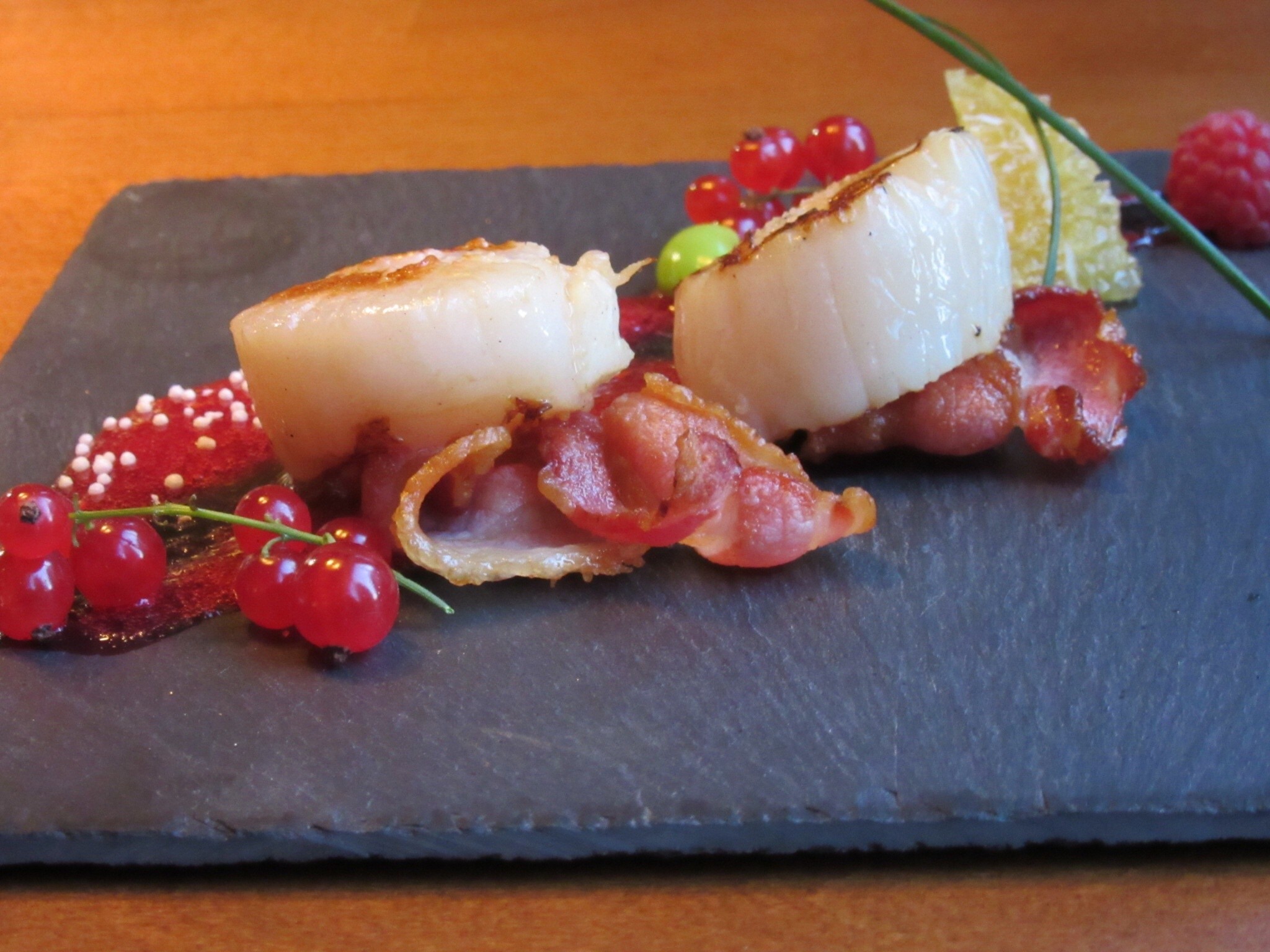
left=1, top=144, right=1269, bottom=871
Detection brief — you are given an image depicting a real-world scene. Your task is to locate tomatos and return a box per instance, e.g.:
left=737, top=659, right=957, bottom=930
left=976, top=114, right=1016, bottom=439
left=655, top=222, right=747, bottom=297
